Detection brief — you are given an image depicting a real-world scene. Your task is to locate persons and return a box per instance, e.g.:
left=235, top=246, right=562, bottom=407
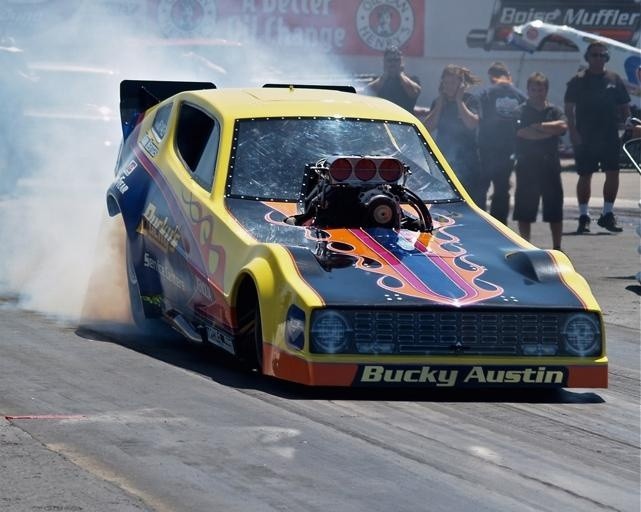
left=631, top=20, right=641, bottom=50
left=0, top=31, right=40, bottom=201
left=510, top=69, right=568, bottom=251
left=469, top=61, right=528, bottom=226
left=370, top=6, right=396, bottom=37
left=366, top=43, right=423, bottom=114
left=421, top=61, right=483, bottom=205
left=563, top=40, right=631, bottom=233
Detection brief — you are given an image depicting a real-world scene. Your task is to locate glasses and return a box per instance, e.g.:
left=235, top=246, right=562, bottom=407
left=586, top=51, right=606, bottom=57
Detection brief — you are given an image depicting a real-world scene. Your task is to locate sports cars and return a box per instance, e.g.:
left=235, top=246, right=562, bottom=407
left=103, top=79, right=609, bottom=390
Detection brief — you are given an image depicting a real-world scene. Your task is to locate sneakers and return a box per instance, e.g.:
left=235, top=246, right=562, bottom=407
left=577, top=214, right=591, bottom=232
left=596, top=212, right=623, bottom=232
